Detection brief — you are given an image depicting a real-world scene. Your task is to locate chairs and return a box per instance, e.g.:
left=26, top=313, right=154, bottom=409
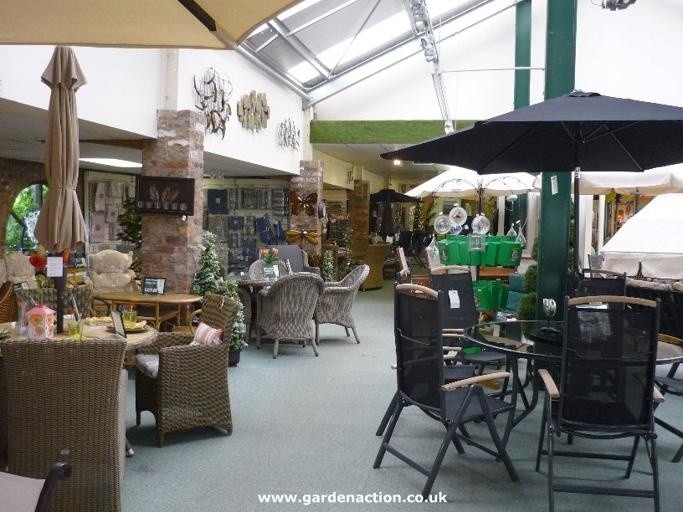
left=0, top=229, right=683, bottom=512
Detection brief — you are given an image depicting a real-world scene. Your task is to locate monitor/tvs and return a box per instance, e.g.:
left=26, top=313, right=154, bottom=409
left=135, top=175, right=194, bottom=216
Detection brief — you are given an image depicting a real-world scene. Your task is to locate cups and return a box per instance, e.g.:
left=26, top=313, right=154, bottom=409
left=66, top=322, right=84, bottom=341
left=123, top=309, right=137, bottom=328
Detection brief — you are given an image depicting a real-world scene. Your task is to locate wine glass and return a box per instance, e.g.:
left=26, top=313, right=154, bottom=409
left=539, top=298, right=557, bottom=333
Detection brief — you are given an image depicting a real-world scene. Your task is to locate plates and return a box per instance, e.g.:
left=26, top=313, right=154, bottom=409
left=124, top=327, right=145, bottom=332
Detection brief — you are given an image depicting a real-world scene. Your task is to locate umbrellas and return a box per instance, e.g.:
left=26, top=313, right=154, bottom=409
left=533, top=163, right=682, bottom=214
left=405, top=166, right=535, bottom=216
left=598, top=192, right=683, bottom=279
left=0, top=0, right=302, bottom=49
left=41, top=46, right=86, bottom=336
left=380, top=88, right=683, bottom=299
